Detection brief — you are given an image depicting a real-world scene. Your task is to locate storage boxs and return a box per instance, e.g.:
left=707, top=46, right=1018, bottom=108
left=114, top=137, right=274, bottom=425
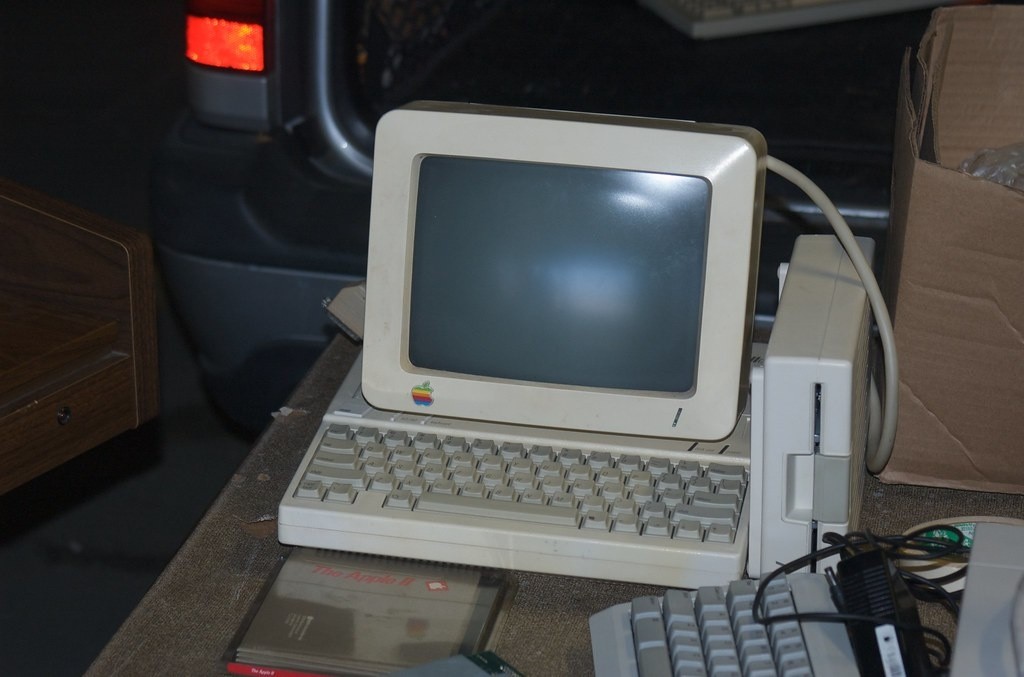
left=873, top=6, right=1023, bottom=496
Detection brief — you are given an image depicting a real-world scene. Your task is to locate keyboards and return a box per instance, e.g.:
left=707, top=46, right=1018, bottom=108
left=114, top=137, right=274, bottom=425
left=293, top=423, right=748, bottom=547
left=590, top=570, right=860, bottom=677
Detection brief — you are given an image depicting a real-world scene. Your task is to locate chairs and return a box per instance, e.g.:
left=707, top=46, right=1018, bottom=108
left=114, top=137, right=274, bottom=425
left=0, top=179, right=161, bottom=492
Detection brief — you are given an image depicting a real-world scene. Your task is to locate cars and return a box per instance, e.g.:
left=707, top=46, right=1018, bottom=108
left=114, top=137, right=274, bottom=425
left=140, top=0, right=947, bottom=447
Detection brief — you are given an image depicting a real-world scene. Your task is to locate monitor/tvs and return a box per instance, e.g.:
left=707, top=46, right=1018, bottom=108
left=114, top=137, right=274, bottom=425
left=360, top=100, right=767, bottom=440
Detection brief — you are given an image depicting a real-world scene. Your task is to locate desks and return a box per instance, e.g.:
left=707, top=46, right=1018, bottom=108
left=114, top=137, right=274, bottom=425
left=84, top=333, right=1024, bottom=677
